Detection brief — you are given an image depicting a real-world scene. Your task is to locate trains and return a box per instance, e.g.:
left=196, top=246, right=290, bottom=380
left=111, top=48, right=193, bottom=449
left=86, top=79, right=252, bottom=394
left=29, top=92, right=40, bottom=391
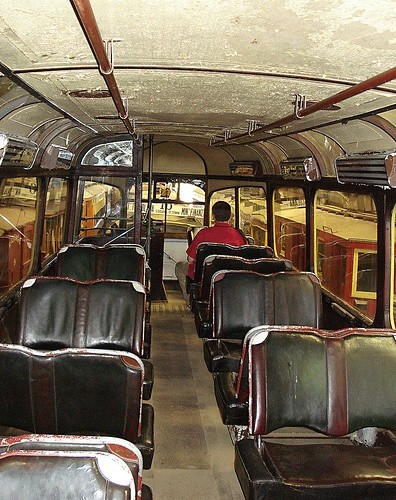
left=213, top=192, right=396, bottom=331
left=1, top=180, right=115, bottom=298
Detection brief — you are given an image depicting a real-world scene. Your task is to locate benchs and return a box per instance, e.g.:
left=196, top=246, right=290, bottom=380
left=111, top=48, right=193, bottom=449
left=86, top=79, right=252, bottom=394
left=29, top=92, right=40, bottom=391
left=214, top=324, right=276, bottom=424
left=56, top=244, right=152, bottom=359
left=234, top=326, right=396, bottom=500
left=0, top=433, right=144, bottom=500
left=193, top=269, right=226, bottom=339
left=204, top=270, right=324, bottom=373
left=185, top=241, right=275, bottom=294
left=189, top=254, right=293, bottom=314
left=0, top=343, right=153, bottom=500
left=18, top=276, right=153, bottom=401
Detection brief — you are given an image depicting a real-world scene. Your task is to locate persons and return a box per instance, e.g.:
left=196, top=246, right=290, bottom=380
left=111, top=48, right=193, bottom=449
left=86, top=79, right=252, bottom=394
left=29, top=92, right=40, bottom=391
left=160, top=183, right=172, bottom=210
left=174, top=201, right=250, bottom=309
